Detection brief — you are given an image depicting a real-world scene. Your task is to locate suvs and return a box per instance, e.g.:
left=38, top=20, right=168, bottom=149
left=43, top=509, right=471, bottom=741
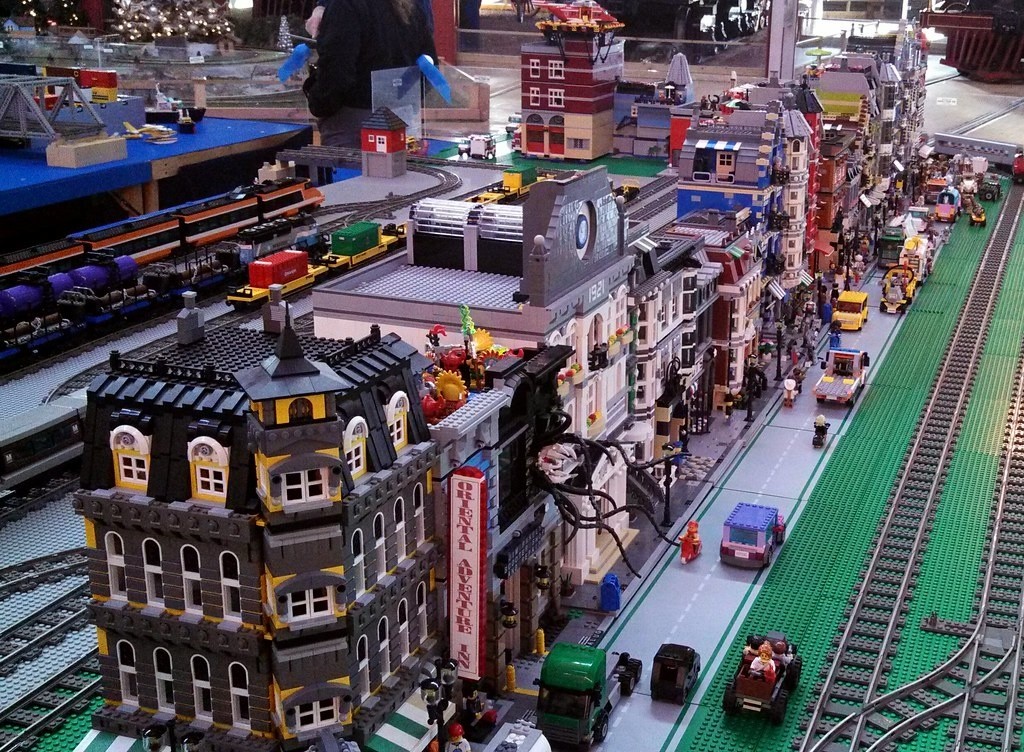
left=830, top=289, right=868, bottom=331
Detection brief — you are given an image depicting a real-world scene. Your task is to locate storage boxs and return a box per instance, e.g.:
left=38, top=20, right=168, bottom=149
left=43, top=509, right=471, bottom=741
left=0, top=61, right=124, bottom=109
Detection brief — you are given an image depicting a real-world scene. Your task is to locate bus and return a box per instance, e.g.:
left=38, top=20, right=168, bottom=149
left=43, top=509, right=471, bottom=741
left=719, top=501, right=788, bottom=570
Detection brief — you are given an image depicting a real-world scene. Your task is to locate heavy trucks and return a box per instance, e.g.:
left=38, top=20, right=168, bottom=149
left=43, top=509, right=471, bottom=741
left=531, top=640, right=644, bottom=752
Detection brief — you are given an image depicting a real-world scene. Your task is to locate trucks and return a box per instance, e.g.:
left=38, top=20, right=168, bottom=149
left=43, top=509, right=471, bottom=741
left=479, top=718, right=552, bottom=752
left=813, top=345, right=870, bottom=406
left=720, top=630, right=803, bottom=725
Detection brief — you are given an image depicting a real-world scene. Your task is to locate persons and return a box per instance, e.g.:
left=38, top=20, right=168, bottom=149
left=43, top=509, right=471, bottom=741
left=444, top=681, right=483, bottom=752
left=890, top=277, right=900, bottom=290
left=784, top=228, right=878, bottom=401
left=303, top=0, right=439, bottom=152
left=661, top=441, right=684, bottom=476
left=678, top=520, right=701, bottom=553
left=813, top=415, right=831, bottom=441
left=745, top=642, right=793, bottom=686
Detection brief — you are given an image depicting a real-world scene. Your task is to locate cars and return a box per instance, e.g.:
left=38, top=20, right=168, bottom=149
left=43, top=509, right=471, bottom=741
left=876, top=153, right=1002, bottom=314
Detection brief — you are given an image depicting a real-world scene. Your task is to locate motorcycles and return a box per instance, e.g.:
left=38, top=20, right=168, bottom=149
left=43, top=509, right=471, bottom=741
left=812, top=422, right=831, bottom=449
left=679, top=537, right=703, bottom=565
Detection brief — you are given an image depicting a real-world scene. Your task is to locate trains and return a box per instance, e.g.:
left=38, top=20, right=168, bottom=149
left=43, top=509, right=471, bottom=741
left=225, top=161, right=561, bottom=313
left=0, top=170, right=324, bottom=288
left=0, top=210, right=324, bottom=364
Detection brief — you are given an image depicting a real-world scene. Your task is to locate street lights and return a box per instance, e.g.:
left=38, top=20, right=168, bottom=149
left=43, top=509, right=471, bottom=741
left=420, top=657, right=458, bottom=752
left=841, top=232, right=853, bottom=290
left=815, top=269, right=824, bottom=319
left=880, top=200, right=888, bottom=226
left=743, top=352, right=758, bottom=423
left=659, top=442, right=676, bottom=528
left=873, top=211, right=881, bottom=256
left=774, top=317, right=786, bottom=382
left=892, top=189, right=900, bottom=216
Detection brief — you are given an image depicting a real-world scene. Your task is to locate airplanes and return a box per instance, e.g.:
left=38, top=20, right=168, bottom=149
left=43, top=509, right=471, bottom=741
left=119, top=82, right=195, bottom=144
